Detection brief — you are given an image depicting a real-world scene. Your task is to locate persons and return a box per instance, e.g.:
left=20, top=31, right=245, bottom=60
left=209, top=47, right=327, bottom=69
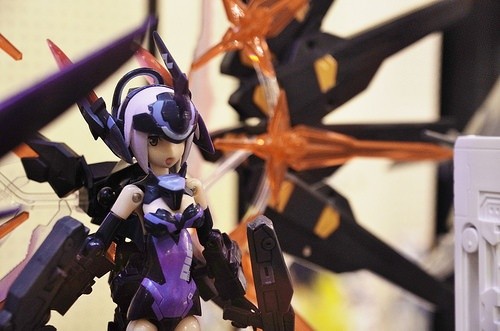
left=83, top=83, right=254, bottom=331
